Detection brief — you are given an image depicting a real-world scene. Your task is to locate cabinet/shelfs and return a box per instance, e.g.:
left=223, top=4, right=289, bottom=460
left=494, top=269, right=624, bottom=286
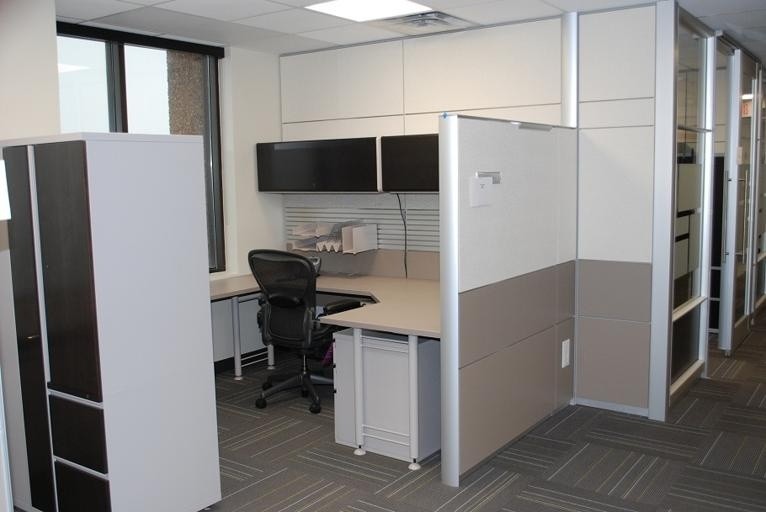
left=0, top=131, right=222, bottom=511
left=333, top=327, right=442, bottom=460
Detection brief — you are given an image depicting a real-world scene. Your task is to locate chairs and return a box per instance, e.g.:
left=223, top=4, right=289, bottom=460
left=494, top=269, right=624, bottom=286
left=246, top=248, right=361, bottom=413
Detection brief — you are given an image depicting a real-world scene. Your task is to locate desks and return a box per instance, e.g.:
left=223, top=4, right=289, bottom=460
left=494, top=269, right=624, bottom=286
left=210, top=271, right=442, bottom=469
